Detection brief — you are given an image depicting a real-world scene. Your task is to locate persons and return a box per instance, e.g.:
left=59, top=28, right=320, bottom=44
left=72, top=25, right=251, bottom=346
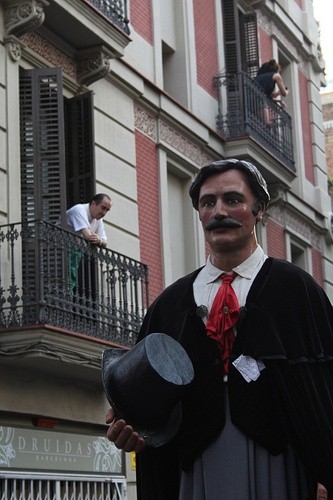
left=46, top=192, right=113, bottom=314
left=105, top=158, right=333, bottom=500
left=247, top=58, right=289, bottom=134
